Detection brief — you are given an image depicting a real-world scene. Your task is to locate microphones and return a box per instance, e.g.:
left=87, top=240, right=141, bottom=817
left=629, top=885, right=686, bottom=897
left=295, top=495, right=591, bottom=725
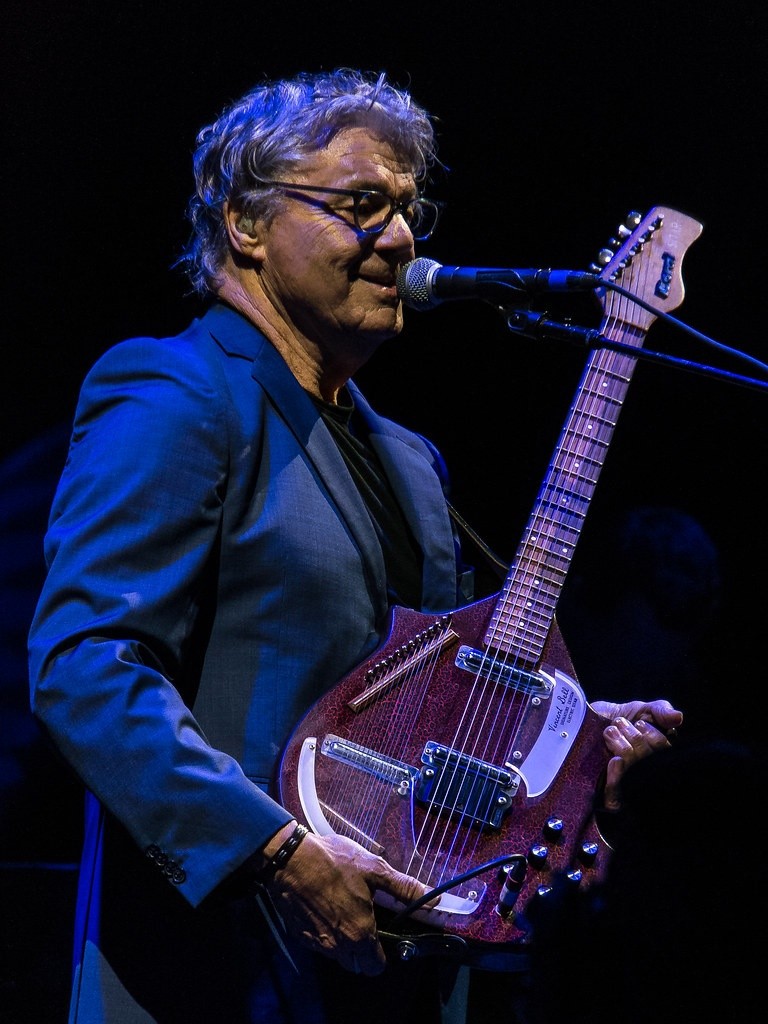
left=396, top=257, right=604, bottom=311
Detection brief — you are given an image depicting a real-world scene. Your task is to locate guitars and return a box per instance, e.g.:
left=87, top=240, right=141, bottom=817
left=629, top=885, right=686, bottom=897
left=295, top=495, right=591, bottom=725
left=278, top=206, right=709, bottom=945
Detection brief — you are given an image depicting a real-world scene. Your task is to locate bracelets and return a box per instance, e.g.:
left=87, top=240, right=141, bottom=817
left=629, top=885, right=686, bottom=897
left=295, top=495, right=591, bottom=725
left=267, top=824, right=308, bottom=871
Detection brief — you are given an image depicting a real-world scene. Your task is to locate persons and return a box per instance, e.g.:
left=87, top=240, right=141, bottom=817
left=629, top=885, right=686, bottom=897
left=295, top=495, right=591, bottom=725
left=28, top=69, right=682, bottom=1024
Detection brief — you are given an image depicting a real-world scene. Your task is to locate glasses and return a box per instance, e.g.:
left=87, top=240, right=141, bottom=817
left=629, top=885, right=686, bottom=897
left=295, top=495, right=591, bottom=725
left=229, top=178, right=440, bottom=241
